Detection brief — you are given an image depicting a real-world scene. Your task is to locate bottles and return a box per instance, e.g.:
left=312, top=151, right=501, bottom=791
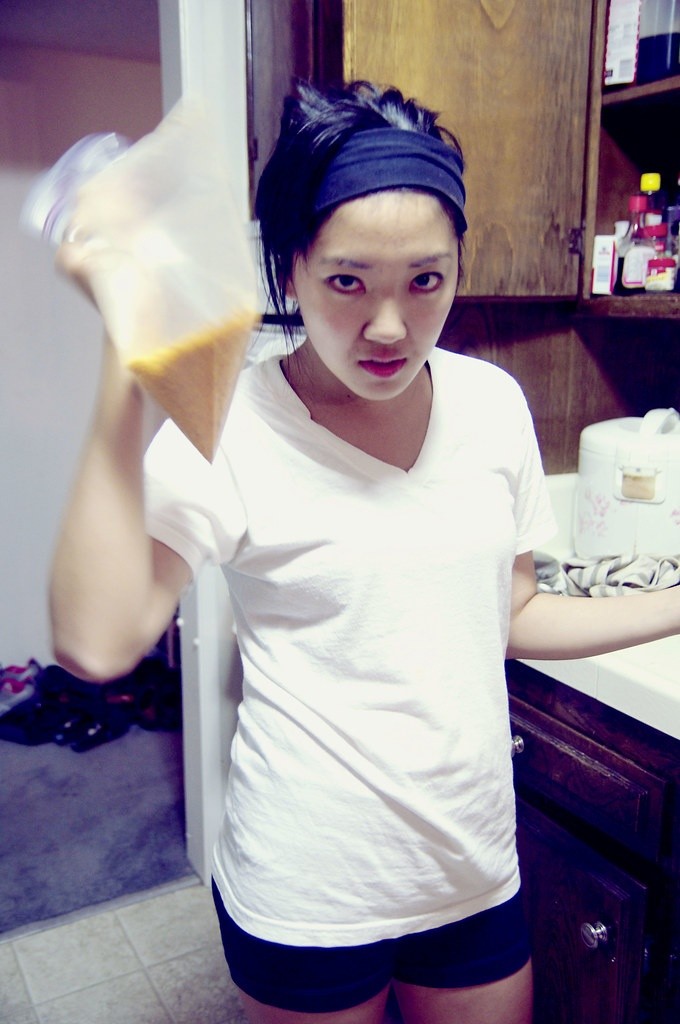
left=613, top=172, right=676, bottom=296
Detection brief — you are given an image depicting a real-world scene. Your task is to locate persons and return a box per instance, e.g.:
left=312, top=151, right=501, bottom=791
left=46, top=78, right=680, bottom=1024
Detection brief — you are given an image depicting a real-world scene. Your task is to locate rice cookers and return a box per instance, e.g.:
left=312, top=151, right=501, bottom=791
left=572, top=408, right=680, bottom=564
